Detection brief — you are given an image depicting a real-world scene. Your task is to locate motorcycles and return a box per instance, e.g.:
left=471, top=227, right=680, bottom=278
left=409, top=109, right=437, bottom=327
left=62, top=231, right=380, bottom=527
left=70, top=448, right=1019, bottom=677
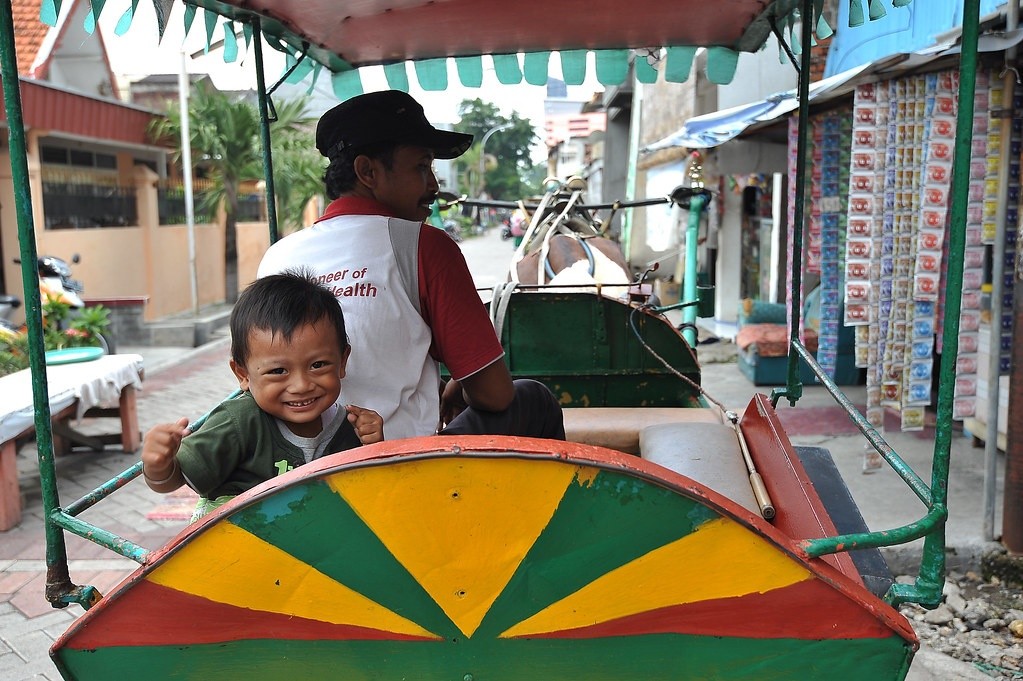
left=0, top=253, right=117, bottom=357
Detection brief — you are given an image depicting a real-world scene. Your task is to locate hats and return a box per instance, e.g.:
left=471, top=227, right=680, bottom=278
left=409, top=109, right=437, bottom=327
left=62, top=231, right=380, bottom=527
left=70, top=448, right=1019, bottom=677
left=316, top=89, right=475, bottom=160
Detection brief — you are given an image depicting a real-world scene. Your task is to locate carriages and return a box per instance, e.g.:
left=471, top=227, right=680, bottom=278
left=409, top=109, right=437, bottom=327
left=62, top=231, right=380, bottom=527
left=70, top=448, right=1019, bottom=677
left=0, top=0, right=983, bottom=681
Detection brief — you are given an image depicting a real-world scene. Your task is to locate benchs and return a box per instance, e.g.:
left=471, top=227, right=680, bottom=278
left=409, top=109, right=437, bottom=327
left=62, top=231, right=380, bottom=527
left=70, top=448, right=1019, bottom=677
left=559, top=397, right=860, bottom=587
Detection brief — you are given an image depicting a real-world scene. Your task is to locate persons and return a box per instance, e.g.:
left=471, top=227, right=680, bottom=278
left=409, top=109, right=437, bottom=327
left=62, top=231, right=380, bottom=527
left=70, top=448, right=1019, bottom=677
left=255, top=90, right=567, bottom=441
left=142, top=274, right=385, bottom=524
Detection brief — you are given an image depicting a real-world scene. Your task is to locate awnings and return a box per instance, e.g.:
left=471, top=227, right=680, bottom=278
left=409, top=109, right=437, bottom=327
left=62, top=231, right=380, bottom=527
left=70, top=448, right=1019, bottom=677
left=637, top=1, right=1023, bottom=157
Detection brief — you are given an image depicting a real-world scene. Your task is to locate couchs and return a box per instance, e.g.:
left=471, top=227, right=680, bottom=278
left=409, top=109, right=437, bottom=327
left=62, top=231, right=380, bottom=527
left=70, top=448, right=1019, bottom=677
left=733, top=284, right=859, bottom=386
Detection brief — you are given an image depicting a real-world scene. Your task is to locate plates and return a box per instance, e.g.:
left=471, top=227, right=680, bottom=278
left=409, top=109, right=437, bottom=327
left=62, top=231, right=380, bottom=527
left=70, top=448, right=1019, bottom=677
left=44, top=346, right=104, bottom=365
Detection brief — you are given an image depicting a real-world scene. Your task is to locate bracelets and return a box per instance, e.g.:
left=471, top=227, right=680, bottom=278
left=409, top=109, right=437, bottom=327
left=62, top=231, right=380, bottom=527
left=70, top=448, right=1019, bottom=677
left=142, top=461, right=176, bottom=485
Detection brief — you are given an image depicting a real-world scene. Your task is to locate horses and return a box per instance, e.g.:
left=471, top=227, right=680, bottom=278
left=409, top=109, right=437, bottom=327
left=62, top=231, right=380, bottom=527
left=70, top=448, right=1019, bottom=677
left=506, top=176, right=637, bottom=305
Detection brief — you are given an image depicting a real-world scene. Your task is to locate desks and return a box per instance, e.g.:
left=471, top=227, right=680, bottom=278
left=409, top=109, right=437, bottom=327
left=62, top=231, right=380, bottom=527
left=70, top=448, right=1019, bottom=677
left=0, top=353, right=145, bottom=533
